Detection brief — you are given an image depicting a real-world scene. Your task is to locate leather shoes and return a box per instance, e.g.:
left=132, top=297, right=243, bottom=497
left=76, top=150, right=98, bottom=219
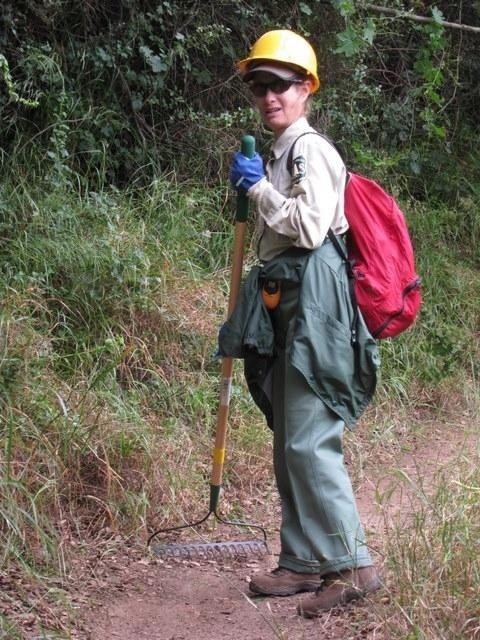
left=296, top=564, right=383, bottom=619
left=246, top=565, right=323, bottom=597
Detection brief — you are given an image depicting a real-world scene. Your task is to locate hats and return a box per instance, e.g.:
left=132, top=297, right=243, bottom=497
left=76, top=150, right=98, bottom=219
left=240, top=62, right=300, bottom=86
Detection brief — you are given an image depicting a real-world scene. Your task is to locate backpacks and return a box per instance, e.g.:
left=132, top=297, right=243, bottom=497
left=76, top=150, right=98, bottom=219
left=286, top=132, right=423, bottom=340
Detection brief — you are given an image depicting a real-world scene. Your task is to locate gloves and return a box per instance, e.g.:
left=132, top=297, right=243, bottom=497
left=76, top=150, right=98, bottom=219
left=228, top=149, right=266, bottom=196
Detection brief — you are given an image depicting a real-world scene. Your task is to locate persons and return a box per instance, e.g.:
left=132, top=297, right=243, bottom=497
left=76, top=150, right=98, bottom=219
left=210, top=28, right=384, bottom=621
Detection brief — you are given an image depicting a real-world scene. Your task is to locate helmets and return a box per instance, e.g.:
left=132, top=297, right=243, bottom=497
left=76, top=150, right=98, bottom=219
left=235, top=29, right=322, bottom=93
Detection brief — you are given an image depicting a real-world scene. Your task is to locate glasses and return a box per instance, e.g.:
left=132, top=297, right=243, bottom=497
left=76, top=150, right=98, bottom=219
left=248, top=80, right=297, bottom=97
